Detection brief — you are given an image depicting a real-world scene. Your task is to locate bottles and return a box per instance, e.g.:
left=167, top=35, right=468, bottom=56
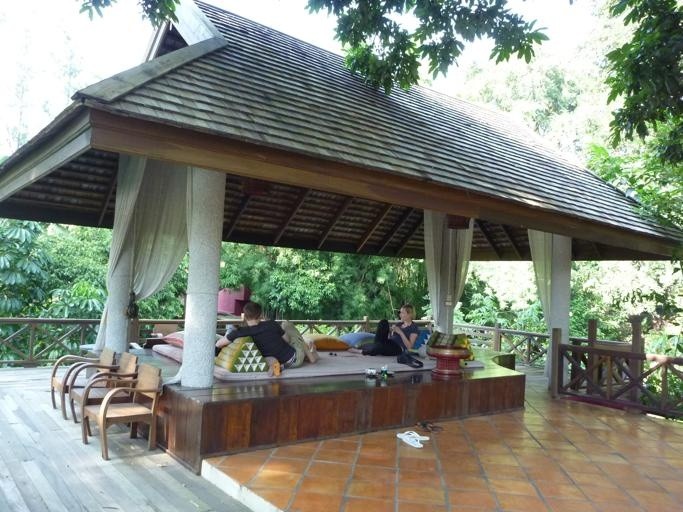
left=380, top=367, right=388, bottom=378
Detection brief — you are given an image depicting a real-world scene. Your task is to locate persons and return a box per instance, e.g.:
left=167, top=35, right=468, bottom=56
left=361, top=304, right=420, bottom=356
left=215, top=301, right=319, bottom=369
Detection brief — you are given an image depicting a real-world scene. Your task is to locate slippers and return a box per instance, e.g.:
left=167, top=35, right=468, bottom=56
left=397, top=431, right=430, bottom=449
left=415, top=421, right=443, bottom=431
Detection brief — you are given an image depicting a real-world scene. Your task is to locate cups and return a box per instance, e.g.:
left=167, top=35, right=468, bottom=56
left=364, top=368, right=377, bottom=378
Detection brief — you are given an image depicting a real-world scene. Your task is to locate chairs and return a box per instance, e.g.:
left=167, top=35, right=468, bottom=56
left=49, top=346, right=162, bottom=461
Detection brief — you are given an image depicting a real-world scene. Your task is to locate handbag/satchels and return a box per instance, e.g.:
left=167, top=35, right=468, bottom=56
left=397, top=352, right=423, bottom=368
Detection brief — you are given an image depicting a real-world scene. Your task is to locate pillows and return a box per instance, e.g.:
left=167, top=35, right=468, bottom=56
left=164, top=331, right=269, bottom=372
left=300, top=327, right=469, bottom=351
